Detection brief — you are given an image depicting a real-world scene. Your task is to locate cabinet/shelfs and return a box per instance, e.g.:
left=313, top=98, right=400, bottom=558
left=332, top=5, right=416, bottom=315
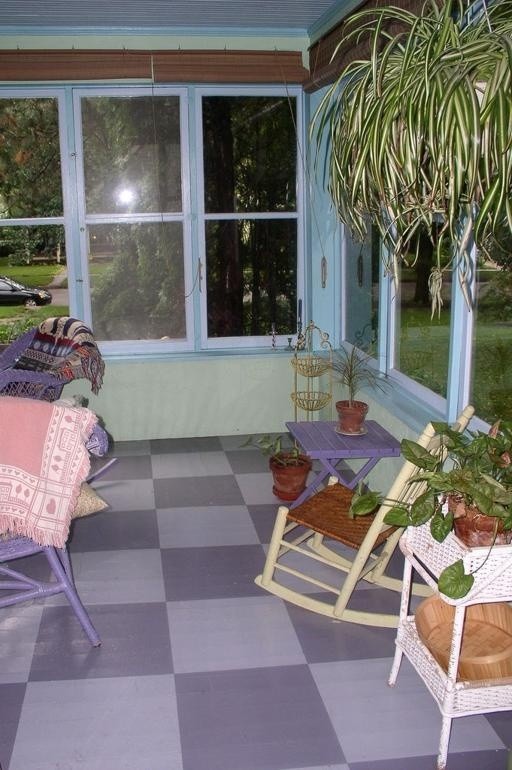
left=291, top=321, right=333, bottom=422
left=387, top=499, right=512, bottom=770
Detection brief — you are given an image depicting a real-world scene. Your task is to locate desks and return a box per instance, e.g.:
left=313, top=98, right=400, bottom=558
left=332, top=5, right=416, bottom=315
left=284, top=419, right=403, bottom=511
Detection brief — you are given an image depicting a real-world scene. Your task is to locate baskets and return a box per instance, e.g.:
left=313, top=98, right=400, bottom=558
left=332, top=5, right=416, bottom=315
left=288, top=355, right=331, bottom=377
left=289, top=391, right=331, bottom=413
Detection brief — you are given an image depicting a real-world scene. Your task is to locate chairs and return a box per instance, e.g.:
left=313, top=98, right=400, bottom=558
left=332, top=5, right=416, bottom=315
left=255, top=406, right=475, bottom=630
left=0, top=314, right=119, bottom=651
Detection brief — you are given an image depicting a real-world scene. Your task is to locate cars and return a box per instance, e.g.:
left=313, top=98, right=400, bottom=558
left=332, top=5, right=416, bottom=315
left=0, top=275, right=53, bottom=306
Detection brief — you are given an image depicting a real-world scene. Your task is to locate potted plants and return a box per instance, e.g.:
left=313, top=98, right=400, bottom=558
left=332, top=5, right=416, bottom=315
left=245, top=338, right=403, bottom=500
left=381, top=419, right=512, bottom=599
